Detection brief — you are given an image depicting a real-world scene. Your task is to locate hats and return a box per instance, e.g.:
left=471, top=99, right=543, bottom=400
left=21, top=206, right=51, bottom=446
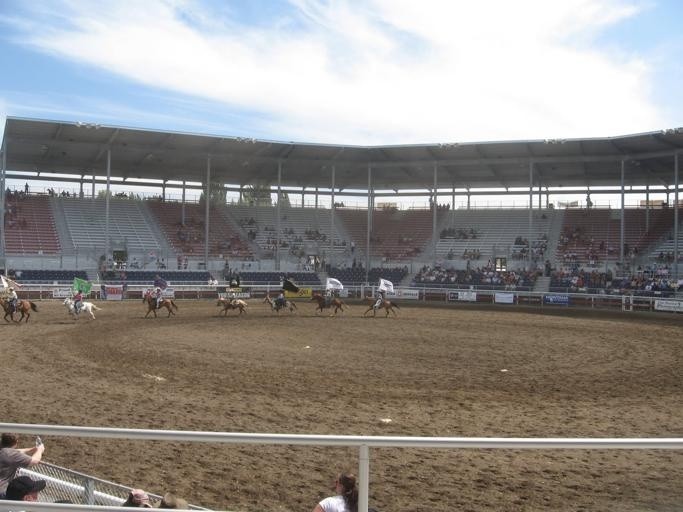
left=129, top=488, right=151, bottom=508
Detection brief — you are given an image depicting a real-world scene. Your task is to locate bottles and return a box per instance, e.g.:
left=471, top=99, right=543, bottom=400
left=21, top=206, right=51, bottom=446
left=35, top=434, right=42, bottom=450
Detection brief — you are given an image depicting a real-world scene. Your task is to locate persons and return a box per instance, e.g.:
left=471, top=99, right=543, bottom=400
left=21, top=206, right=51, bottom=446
left=123, top=489, right=152, bottom=508
left=313, top=472, right=358, bottom=512
left=3, top=182, right=682, bottom=315
left=0, top=433, right=45, bottom=501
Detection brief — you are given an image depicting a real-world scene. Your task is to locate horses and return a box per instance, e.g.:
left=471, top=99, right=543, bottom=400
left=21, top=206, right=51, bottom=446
left=0, top=297, right=38, bottom=324
left=62, top=297, right=103, bottom=320
left=142, top=291, right=178, bottom=318
left=262, top=293, right=298, bottom=319
left=217, top=296, right=248, bottom=317
left=361, top=295, right=401, bottom=318
left=310, top=292, right=349, bottom=316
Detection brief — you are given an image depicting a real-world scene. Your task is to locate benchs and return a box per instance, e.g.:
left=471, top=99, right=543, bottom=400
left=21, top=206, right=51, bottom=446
left=0, top=194, right=682, bottom=285
